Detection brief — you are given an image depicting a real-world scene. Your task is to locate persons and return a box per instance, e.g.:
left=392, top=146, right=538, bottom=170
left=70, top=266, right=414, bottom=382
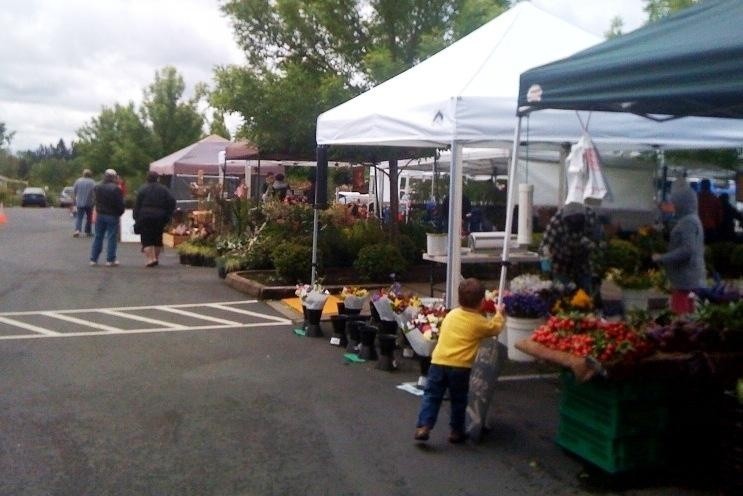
left=73, top=169, right=96, bottom=237
left=652, top=181, right=706, bottom=290
left=443, top=194, right=471, bottom=232
left=90, top=169, right=124, bottom=266
left=414, top=278, right=506, bottom=444
left=263, top=172, right=291, bottom=201
left=695, top=180, right=743, bottom=243
left=540, top=202, right=605, bottom=295
left=133, top=171, right=176, bottom=267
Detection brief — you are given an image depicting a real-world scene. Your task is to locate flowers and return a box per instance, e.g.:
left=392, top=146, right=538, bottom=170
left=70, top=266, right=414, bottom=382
left=294, top=272, right=450, bottom=389
left=483, top=266, right=737, bottom=319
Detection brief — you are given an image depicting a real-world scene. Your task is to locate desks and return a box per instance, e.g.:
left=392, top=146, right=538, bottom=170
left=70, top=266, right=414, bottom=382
left=422, top=251, right=540, bottom=297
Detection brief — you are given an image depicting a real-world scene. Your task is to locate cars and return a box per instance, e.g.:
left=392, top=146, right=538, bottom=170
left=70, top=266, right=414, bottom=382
left=60, top=186, right=77, bottom=206
left=21, top=186, right=48, bottom=207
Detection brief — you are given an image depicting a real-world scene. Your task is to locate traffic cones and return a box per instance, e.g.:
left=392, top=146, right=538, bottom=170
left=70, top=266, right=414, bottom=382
left=91, top=207, right=96, bottom=222
left=0, top=200, right=7, bottom=223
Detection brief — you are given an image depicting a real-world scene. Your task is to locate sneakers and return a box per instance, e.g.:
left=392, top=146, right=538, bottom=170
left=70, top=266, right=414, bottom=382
left=106, top=260, right=119, bottom=266
left=448, top=429, right=462, bottom=441
left=415, top=427, right=429, bottom=440
left=73, top=230, right=94, bottom=237
left=89, top=260, right=96, bottom=266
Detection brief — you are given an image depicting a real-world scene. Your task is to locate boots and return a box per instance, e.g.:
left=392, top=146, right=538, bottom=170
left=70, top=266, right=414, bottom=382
left=144, top=245, right=161, bottom=266
